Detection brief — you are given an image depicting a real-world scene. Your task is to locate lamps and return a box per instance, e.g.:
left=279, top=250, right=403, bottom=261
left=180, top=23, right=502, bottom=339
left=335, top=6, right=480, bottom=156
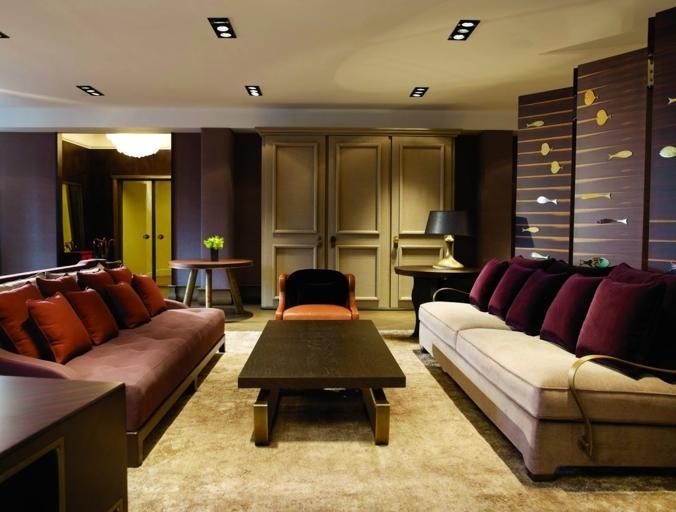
left=425, top=211, right=472, bottom=271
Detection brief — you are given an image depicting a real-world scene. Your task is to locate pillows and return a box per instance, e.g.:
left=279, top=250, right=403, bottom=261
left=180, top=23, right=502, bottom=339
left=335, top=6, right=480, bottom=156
left=556, top=257, right=613, bottom=279
left=109, top=282, right=153, bottom=330
left=576, top=262, right=664, bottom=377
left=0, top=281, right=51, bottom=359
left=66, top=287, right=119, bottom=345
left=487, top=255, right=551, bottom=320
left=104, top=264, right=132, bottom=284
left=37, top=274, right=80, bottom=297
left=78, top=269, right=115, bottom=287
left=469, top=257, right=506, bottom=312
left=509, top=253, right=554, bottom=272
left=610, top=260, right=676, bottom=286
left=538, top=271, right=608, bottom=352
left=134, top=274, right=168, bottom=317
left=25, top=290, right=92, bottom=364
left=505, top=260, right=574, bottom=336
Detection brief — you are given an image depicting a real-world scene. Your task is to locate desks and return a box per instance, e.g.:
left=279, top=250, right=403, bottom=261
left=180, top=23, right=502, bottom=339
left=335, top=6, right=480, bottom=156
left=0, top=376, right=129, bottom=512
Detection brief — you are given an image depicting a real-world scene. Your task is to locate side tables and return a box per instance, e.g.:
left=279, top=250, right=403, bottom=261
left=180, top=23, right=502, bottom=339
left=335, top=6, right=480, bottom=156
left=393, top=265, right=481, bottom=337
left=168, top=257, right=256, bottom=314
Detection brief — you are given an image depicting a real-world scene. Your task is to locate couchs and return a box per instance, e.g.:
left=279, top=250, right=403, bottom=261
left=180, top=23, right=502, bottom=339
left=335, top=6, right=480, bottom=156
left=1, top=258, right=225, bottom=468
left=418, top=254, right=676, bottom=482
left=257, top=127, right=390, bottom=310
left=275, top=268, right=359, bottom=319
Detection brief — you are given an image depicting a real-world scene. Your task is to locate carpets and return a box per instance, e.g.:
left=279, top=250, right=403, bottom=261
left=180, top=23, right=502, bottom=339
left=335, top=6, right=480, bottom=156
left=128, top=326, right=675, bottom=512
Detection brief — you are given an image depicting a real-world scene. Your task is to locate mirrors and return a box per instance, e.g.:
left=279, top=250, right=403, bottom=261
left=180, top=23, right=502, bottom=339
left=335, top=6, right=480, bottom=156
left=61, top=181, right=87, bottom=253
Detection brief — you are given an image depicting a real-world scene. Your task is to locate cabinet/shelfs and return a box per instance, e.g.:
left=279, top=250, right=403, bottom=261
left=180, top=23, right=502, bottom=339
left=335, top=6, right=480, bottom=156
left=112, top=174, right=171, bottom=279
left=390, top=127, right=462, bottom=311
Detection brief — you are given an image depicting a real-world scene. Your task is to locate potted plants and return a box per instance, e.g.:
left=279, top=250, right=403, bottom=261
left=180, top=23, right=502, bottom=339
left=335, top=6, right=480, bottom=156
left=204, top=236, right=225, bottom=260
